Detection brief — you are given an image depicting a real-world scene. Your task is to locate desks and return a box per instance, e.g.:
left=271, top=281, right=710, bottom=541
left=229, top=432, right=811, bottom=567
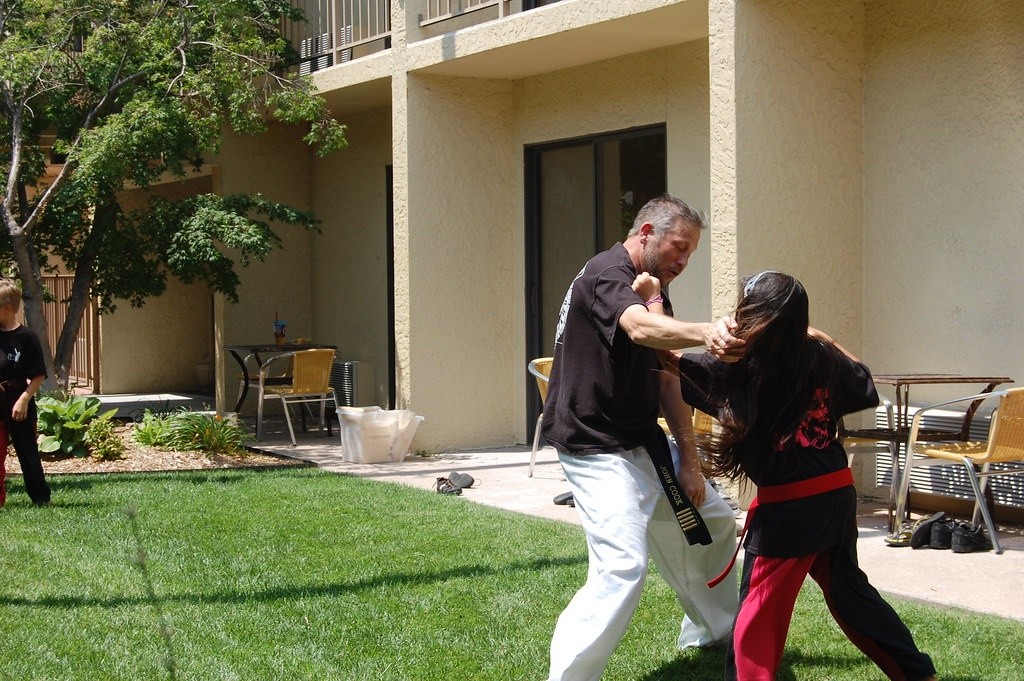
left=221, top=343, right=338, bottom=437
left=835, top=373, right=1013, bottom=533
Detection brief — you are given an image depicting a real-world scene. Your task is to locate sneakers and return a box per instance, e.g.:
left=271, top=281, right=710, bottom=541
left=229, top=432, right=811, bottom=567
left=951, top=524, right=992, bottom=552
left=930, top=520, right=958, bottom=548
left=449, top=471, right=474, bottom=487
left=437, top=477, right=462, bottom=495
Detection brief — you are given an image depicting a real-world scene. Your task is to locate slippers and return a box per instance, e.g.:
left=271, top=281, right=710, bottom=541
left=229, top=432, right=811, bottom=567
left=884, top=526, right=911, bottom=546
left=910, top=512, right=944, bottom=549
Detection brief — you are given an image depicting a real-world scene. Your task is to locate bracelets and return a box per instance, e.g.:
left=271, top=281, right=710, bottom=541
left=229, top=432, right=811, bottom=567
left=646, top=294, right=664, bottom=305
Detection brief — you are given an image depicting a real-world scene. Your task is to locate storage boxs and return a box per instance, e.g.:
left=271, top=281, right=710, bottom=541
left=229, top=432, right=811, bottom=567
left=335, top=405, right=425, bottom=464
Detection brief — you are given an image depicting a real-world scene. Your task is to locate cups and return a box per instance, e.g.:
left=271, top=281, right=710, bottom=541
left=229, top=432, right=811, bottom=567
left=273, top=320, right=288, bottom=346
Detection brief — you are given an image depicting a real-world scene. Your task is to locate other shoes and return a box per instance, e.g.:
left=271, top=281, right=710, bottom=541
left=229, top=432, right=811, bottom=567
left=554, top=491, right=574, bottom=504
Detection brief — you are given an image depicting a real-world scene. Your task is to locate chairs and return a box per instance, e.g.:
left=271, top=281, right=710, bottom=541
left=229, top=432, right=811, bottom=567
left=236, top=337, right=313, bottom=423
left=656, top=407, right=712, bottom=482
left=842, top=436, right=903, bottom=530
left=528, top=357, right=553, bottom=479
left=262, top=348, right=340, bottom=446
left=892, top=387, right=1024, bottom=555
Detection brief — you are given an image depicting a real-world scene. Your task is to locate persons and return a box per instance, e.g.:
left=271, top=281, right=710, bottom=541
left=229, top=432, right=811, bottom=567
left=0, top=279, right=52, bottom=505
left=631, top=272, right=937, bottom=681
left=540, top=195, right=737, bottom=681
